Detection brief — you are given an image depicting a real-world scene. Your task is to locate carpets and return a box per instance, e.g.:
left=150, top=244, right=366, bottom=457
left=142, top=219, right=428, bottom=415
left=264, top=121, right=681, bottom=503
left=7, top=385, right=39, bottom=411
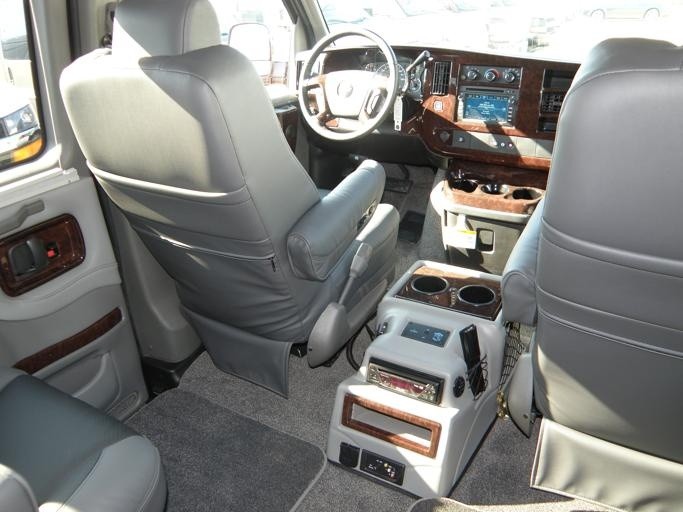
left=122, top=386, right=327, bottom=512
left=408, top=496, right=483, bottom=512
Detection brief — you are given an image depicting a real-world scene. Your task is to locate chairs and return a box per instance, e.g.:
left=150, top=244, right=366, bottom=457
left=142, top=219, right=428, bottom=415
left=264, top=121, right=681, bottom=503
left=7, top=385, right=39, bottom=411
left=0, top=361, right=173, bottom=511
left=500, top=39, right=683, bottom=512
left=59, top=0, right=401, bottom=402
